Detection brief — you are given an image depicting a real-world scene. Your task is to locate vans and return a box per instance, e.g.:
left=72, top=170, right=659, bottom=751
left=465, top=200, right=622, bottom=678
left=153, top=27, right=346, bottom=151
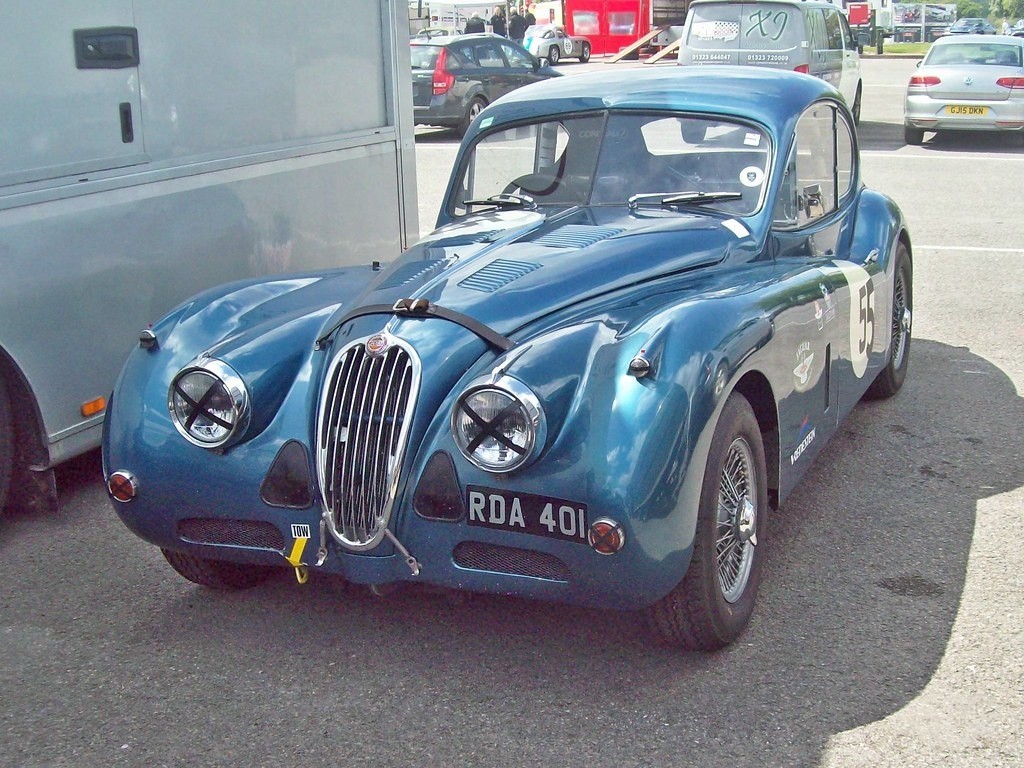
left=676, top=0, right=872, bottom=144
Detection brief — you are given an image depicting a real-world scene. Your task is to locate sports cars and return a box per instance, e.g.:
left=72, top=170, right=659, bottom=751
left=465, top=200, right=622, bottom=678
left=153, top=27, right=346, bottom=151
left=502, top=26, right=592, bottom=63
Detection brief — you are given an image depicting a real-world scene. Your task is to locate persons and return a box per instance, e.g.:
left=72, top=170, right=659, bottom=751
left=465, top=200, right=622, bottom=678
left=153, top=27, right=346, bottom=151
left=491, top=7, right=506, bottom=37
left=1002, top=19, right=1008, bottom=35
left=464, top=12, right=485, bottom=34
left=509, top=7, right=536, bottom=46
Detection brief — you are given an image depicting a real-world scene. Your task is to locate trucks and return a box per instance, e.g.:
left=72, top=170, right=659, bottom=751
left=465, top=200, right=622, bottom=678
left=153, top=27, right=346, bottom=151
left=1, top=1, right=416, bottom=515
left=649, top=0, right=895, bottom=59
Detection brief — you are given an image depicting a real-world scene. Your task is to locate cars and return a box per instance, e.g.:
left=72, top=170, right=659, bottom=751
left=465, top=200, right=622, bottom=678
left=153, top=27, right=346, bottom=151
left=409, top=25, right=468, bottom=59
left=903, top=35, right=1024, bottom=145
left=1004, top=18, right=1024, bottom=37
left=944, top=18, right=996, bottom=36
left=101, top=63, right=912, bottom=655
left=928, top=6, right=951, bottom=21
left=904, top=9, right=920, bottom=21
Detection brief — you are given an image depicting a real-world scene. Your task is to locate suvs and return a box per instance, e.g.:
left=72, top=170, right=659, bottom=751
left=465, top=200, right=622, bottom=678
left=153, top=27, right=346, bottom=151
left=412, top=31, right=567, bottom=136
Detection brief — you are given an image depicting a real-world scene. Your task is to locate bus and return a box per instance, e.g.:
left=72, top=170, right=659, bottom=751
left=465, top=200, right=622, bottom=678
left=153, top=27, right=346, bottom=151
left=431, top=13, right=470, bottom=26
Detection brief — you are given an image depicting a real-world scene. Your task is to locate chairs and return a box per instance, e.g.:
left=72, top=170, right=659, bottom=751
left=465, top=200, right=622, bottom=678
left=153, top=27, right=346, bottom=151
left=690, top=126, right=784, bottom=209
left=542, top=120, right=664, bottom=205
left=996, top=50, right=1017, bottom=62
